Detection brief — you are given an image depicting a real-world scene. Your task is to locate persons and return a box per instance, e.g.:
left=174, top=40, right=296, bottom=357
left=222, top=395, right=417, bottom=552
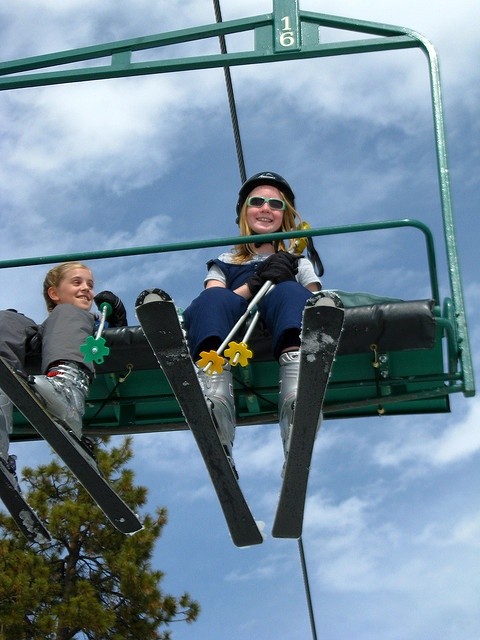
left=0, top=261, right=129, bottom=495
left=182, top=171, right=326, bottom=461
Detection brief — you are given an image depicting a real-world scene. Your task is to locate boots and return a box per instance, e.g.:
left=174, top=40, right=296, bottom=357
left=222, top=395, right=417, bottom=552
left=1, top=391, right=14, bottom=463
left=28, top=360, right=92, bottom=442
left=278, top=349, right=323, bottom=458
left=199, top=370, right=236, bottom=458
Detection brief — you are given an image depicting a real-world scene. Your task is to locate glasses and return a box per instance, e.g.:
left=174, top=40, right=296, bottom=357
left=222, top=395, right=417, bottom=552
left=247, top=196, right=286, bottom=211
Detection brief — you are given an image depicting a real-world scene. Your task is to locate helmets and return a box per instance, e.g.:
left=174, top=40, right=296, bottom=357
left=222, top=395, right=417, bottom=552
left=237, top=171, right=295, bottom=223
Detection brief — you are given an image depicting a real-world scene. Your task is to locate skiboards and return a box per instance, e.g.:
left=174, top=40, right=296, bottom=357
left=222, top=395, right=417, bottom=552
left=134, top=289, right=345, bottom=549
left=0, top=358, right=145, bottom=545
